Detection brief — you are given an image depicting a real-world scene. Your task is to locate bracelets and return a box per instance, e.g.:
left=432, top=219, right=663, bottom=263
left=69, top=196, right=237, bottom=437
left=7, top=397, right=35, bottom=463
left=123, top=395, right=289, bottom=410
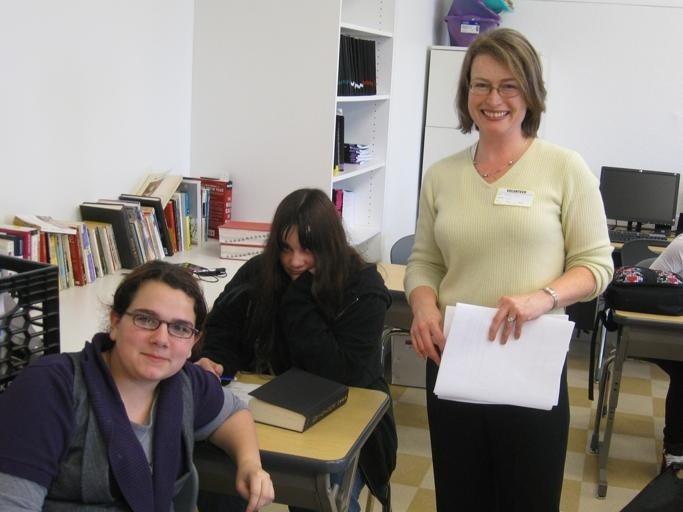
left=542, top=286, right=558, bottom=310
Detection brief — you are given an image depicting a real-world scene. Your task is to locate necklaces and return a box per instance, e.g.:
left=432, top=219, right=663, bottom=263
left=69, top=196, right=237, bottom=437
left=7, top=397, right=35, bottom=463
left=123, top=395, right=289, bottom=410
left=474, top=139, right=529, bottom=177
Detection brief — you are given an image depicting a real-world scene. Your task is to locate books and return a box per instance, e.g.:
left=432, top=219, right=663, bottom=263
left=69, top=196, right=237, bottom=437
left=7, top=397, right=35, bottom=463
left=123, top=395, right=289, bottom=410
left=334, top=35, right=380, bottom=177
left=246, top=365, right=349, bottom=433
left=0, top=176, right=278, bottom=298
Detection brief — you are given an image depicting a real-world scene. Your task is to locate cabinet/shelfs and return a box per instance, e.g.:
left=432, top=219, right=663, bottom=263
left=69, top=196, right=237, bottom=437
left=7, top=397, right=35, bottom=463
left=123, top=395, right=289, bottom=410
left=190, top=0, right=398, bottom=265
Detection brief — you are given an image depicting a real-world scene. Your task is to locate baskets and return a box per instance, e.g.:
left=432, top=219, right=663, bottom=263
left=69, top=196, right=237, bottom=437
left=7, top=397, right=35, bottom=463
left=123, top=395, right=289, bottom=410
left=0, top=254, right=60, bottom=393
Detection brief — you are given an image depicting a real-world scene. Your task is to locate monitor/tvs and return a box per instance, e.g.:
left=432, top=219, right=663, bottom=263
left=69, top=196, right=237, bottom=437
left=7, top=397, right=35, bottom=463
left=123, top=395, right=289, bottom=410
left=599, top=166, right=680, bottom=232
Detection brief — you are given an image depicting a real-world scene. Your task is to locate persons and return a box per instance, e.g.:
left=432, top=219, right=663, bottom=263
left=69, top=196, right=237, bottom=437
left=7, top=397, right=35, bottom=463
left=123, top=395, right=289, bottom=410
left=195, top=190, right=398, bottom=512
left=406, top=28, right=616, bottom=512
left=0, top=258, right=276, bottom=512
left=634, top=234, right=683, bottom=480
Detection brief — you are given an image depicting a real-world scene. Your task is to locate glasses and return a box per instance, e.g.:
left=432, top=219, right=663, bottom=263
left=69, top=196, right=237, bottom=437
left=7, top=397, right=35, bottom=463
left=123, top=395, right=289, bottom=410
left=121, top=311, right=199, bottom=338
left=464, top=78, right=521, bottom=97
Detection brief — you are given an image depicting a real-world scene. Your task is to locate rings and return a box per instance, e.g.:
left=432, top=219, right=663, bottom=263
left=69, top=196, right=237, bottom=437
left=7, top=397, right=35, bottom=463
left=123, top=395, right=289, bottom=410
left=507, top=315, right=514, bottom=323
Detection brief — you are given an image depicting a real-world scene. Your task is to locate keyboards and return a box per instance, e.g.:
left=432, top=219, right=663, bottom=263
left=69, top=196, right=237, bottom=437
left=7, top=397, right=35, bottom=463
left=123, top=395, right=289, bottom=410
left=608, top=230, right=669, bottom=247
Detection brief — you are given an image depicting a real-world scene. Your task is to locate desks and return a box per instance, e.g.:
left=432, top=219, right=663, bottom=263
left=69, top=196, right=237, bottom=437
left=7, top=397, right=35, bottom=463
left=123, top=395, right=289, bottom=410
left=610, top=224, right=676, bottom=255
left=597, top=307, right=683, bottom=498
left=374, top=262, right=407, bottom=293
left=13, top=237, right=248, bottom=354
left=194, top=371, right=392, bottom=511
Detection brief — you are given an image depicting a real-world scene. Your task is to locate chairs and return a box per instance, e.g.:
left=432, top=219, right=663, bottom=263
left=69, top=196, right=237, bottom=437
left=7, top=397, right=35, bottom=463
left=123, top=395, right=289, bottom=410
left=595, top=239, right=672, bottom=381
left=376, top=234, right=416, bottom=379
left=590, top=256, right=672, bottom=454
